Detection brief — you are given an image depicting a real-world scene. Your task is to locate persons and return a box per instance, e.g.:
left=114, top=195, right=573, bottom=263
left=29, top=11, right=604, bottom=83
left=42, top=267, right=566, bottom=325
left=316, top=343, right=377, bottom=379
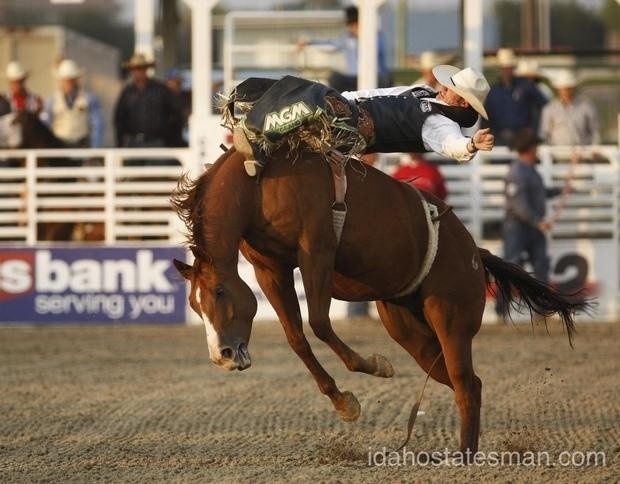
left=492, top=128, right=576, bottom=325
left=480, top=48, right=548, bottom=146
left=37, top=59, right=107, bottom=165
left=534, top=67, right=603, bottom=146
left=297, top=7, right=391, bottom=90
left=110, top=52, right=184, bottom=165
left=225, top=63, right=496, bottom=177
left=387, top=152, right=448, bottom=200
left=0, top=59, right=44, bottom=115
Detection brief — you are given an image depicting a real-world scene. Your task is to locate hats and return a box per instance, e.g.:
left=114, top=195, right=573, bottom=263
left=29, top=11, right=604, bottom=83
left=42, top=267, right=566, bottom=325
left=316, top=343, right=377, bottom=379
left=432, top=64, right=491, bottom=121
left=509, top=128, right=545, bottom=152
left=49, top=59, right=86, bottom=81
left=486, top=49, right=520, bottom=68
left=551, top=69, right=582, bottom=91
left=412, top=51, right=443, bottom=73
left=6, top=62, right=29, bottom=84
left=118, top=55, right=156, bottom=71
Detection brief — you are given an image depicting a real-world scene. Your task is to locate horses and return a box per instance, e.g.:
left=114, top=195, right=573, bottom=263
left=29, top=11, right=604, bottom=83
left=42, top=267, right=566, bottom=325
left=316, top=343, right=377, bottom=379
left=166, top=137, right=602, bottom=465
left=0, top=93, right=80, bottom=241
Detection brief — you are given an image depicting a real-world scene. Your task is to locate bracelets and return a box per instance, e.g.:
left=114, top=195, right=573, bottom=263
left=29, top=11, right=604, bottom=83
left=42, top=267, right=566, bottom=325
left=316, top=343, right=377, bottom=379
left=470, top=136, right=479, bottom=151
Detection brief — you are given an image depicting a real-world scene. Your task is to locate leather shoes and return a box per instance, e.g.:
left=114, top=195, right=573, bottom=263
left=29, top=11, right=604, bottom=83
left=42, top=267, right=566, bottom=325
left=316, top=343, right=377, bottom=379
left=232, top=126, right=270, bottom=176
